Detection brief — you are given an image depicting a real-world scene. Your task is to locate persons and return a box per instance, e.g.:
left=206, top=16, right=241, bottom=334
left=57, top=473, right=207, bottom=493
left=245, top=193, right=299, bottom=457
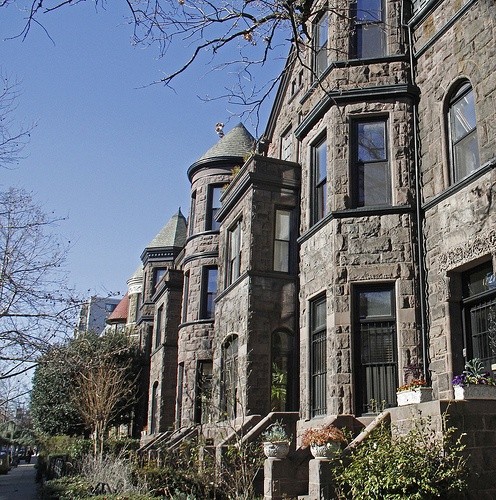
left=27, top=447, right=34, bottom=464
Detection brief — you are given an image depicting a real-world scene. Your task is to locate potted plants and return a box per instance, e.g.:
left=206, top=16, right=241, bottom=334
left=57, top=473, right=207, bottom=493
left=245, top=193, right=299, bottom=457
left=261, top=418, right=293, bottom=460
left=302, top=423, right=346, bottom=460
left=361, top=398, right=387, bottom=416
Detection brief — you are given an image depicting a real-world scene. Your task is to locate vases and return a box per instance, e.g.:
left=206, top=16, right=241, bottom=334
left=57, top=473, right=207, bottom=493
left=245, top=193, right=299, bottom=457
left=453, top=384, right=496, bottom=400
left=396, top=387, right=434, bottom=406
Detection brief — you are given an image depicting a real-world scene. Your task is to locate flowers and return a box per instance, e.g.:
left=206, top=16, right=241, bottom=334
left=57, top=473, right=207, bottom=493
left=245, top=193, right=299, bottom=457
left=397, top=360, right=429, bottom=391
left=451, top=357, right=496, bottom=387
